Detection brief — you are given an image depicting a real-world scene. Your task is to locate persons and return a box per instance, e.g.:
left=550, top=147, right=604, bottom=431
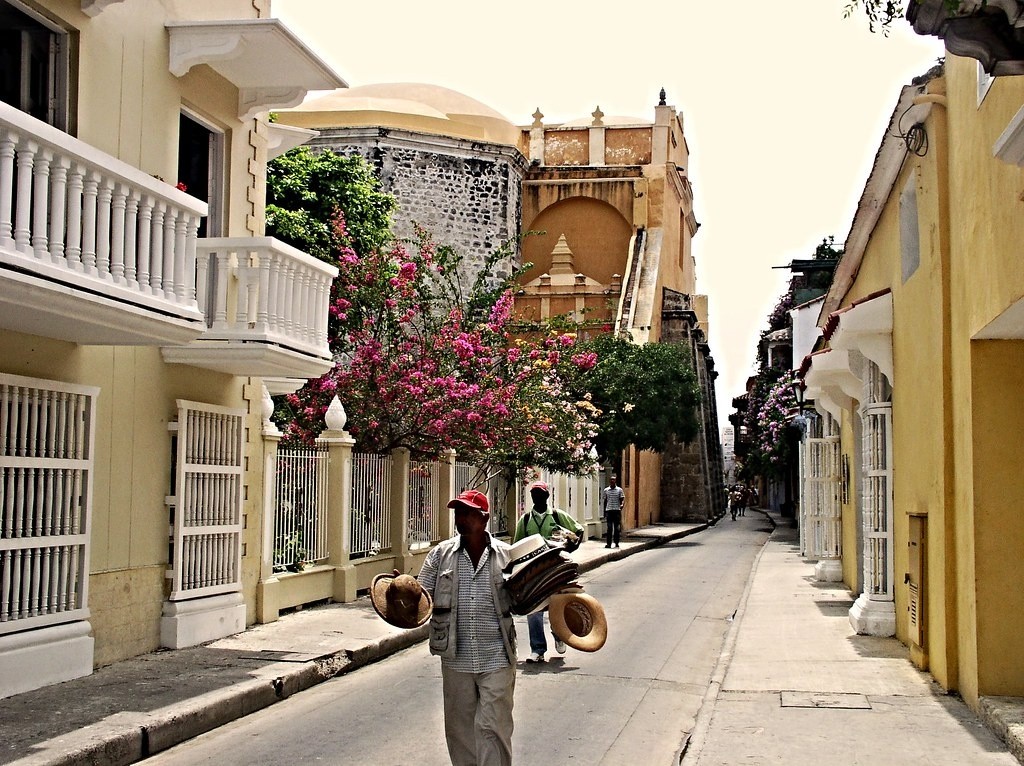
left=603, top=473, right=625, bottom=548
left=724, top=484, right=749, bottom=521
left=393, top=490, right=519, bottom=766
left=514, top=481, right=584, bottom=663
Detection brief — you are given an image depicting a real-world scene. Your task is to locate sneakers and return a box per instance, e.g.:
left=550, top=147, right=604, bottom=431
left=526, top=653, right=544, bottom=663
left=555, top=640, right=566, bottom=654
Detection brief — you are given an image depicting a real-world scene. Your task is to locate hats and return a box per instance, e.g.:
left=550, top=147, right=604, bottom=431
left=447, top=490, right=489, bottom=512
left=502, top=533, right=585, bottom=616
left=529, top=481, right=548, bottom=492
left=549, top=591, right=607, bottom=652
left=371, top=573, right=433, bottom=629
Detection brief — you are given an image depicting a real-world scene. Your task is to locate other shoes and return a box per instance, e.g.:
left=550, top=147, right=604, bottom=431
left=616, top=543, right=619, bottom=547
left=605, top=544, right=611, bottom=548
